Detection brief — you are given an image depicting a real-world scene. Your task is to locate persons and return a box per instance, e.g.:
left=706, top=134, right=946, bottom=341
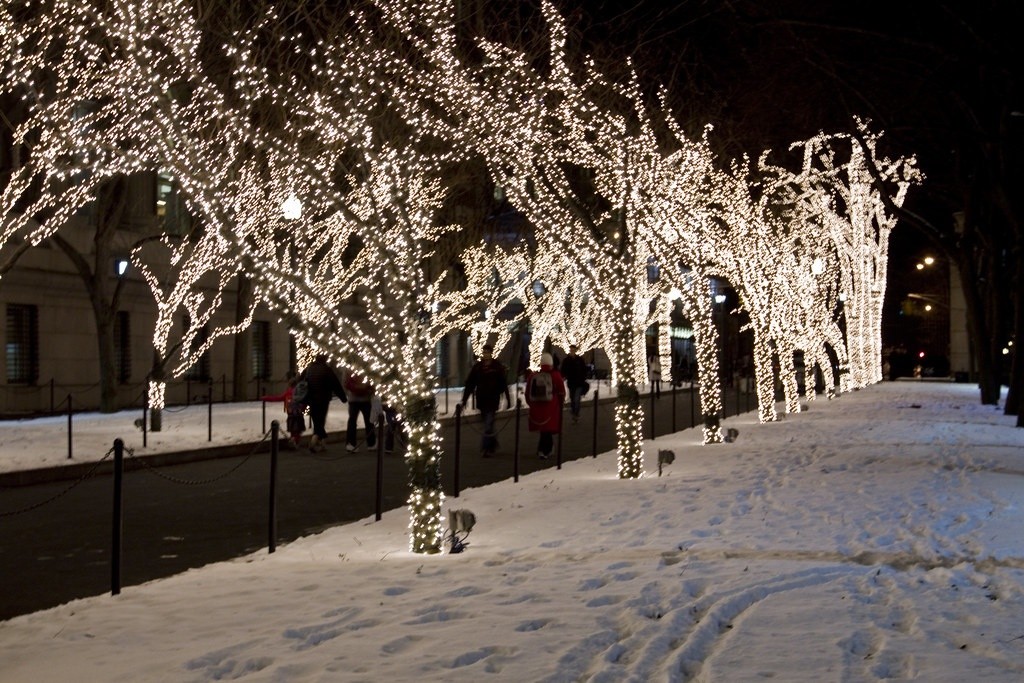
left=648, top=351, right=661, bottom=391
left=461, top=346, right=511, bottom=457
left=563, top=345, right=588, bottom=424
left=261, top=353, right=409, bottom=453
left=525, top=353, right=566, bottom=459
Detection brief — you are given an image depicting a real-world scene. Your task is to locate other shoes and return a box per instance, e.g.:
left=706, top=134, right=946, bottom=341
left=346, top=443, right=360, bottom=452
left=292, top=437, right=302, bottom=445
left=367, top=438, right=378, bottom=450
left=310, top=435, right=325, bottom=451
left=384, top=448, right=392, bottom=452
left=538, top=451, right=548, bottom=461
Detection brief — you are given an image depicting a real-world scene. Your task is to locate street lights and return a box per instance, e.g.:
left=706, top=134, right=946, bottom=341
left=282, top=193, right=302, bottom=382
left=715, top=292, right=727, bottom=379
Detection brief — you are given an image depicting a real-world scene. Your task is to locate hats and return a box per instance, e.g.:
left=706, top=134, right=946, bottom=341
left=540, top=353, right=553, bottom=366
left=481, top=345, right=493, bottom=352
left=569, top=345, right=578, bottom=349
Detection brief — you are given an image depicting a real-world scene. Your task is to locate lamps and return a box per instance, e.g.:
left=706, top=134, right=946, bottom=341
left=447, top=509, right=476, bottom=555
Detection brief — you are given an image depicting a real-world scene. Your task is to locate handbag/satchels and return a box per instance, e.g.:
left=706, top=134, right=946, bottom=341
left=579, top=381, right=589, bottom=395
left=292, top=364, right=310, bottom=401
left=652, top=356, right=661, bottom=374
left=344, top=373, right=375, bottom=397
left=288, top=398, right=305, bottom=412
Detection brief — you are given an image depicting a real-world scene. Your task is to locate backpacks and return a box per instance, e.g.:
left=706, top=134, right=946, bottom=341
left=529, top=372, right=553, bottom=403
